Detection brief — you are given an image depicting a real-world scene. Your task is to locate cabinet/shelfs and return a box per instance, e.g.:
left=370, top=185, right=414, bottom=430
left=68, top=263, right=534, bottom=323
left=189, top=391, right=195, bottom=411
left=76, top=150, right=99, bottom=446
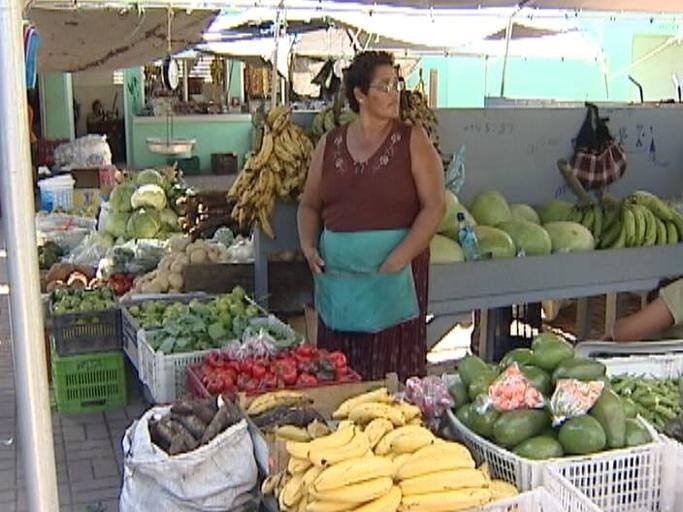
left=87, top=120, right=125, bottom=163
left=37, top=70, right=78, bottom=142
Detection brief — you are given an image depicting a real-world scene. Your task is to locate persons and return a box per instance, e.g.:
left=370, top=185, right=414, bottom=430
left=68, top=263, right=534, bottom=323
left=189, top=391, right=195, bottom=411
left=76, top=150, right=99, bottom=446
left=295, top=50, right=445, bottom=384
left=85, top=99, right=105, bottom=128
left=599, top=277, right=683, bottom=341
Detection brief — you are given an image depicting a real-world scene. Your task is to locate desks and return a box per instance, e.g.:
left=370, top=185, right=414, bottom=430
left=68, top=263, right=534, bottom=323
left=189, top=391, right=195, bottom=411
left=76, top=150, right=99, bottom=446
left=222, top=349, right=679, bottom=512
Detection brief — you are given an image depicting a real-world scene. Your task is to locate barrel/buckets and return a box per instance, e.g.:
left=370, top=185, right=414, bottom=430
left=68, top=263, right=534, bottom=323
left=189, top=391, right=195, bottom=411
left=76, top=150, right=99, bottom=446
left=37, top=174, right=75, bottom=212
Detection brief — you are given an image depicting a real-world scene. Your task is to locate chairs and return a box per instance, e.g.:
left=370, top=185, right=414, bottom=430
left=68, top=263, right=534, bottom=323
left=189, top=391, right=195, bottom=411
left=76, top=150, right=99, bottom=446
left=573, top=338, right=683, bottom=358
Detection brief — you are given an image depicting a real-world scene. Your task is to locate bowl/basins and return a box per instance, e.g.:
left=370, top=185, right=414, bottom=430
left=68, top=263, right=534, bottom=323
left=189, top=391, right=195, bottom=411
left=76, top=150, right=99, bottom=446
left=145, top=138, right=195, bottom=156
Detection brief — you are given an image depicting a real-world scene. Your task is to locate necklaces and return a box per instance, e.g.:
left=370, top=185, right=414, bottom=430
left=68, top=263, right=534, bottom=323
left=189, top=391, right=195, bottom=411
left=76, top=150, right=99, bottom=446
left=350, top=138, right=384, bottom=173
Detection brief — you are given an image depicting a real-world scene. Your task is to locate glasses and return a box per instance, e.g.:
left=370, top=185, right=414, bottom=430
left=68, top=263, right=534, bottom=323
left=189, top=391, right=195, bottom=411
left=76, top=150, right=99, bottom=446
left=370, top=81, right=404, bottom=91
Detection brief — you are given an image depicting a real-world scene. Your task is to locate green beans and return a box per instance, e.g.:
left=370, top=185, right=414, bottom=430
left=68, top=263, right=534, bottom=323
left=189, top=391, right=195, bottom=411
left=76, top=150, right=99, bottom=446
left=609, top=367, right=682, bottom=435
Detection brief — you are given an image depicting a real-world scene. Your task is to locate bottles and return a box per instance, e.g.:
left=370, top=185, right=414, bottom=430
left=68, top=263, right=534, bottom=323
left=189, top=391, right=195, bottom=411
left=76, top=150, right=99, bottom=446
left=457, top=211, right=481, bottom=261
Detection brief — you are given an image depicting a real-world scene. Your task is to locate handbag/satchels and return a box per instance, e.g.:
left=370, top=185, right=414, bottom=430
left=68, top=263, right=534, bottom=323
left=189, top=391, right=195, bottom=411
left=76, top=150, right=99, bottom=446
left=572, top=103, right=626, bottom=190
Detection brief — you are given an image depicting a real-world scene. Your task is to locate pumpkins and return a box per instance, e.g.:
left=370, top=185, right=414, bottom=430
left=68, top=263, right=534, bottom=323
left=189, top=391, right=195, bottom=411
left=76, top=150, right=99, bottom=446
left=83, top=168, right=177, bottom=249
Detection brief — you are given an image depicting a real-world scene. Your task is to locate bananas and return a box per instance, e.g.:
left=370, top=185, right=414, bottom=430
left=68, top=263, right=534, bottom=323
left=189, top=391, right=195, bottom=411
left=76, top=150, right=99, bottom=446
left=569, top=189, right=683, bottom=248
left=402, top=92, right=444, bottom=151
left=311, top=107, right=350, bottom=135
left=244, top=386, right=516, bottom=512
left=223, top=104, right=314, bottom=239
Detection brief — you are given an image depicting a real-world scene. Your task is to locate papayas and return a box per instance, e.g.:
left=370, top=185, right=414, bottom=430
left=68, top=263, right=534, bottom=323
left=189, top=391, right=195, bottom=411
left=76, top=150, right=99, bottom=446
left=451, top=331, right=651, bottom=460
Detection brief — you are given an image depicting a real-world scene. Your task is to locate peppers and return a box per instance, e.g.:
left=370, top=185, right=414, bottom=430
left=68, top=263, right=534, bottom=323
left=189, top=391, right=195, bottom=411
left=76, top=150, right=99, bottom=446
left=200, top=348, right=354, bottom=392
